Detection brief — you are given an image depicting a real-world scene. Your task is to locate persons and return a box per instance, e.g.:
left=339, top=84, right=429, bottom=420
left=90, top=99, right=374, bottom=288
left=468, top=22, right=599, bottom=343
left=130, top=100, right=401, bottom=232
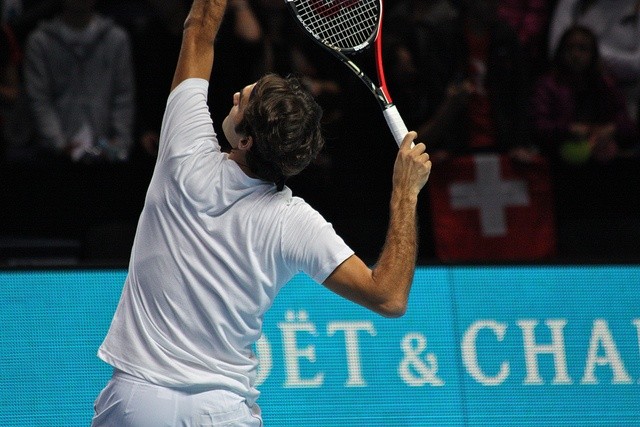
left=415, top=0, right=546, bottom=169
left=21, top=1, right=135, bottom=257
left=2, top=20, right=22, bottom=106
left=531, top=22, right=634, bottom=171
left=90, top=0, right=433, bottom=426
left=228, top=2, right=356, bottom=107
